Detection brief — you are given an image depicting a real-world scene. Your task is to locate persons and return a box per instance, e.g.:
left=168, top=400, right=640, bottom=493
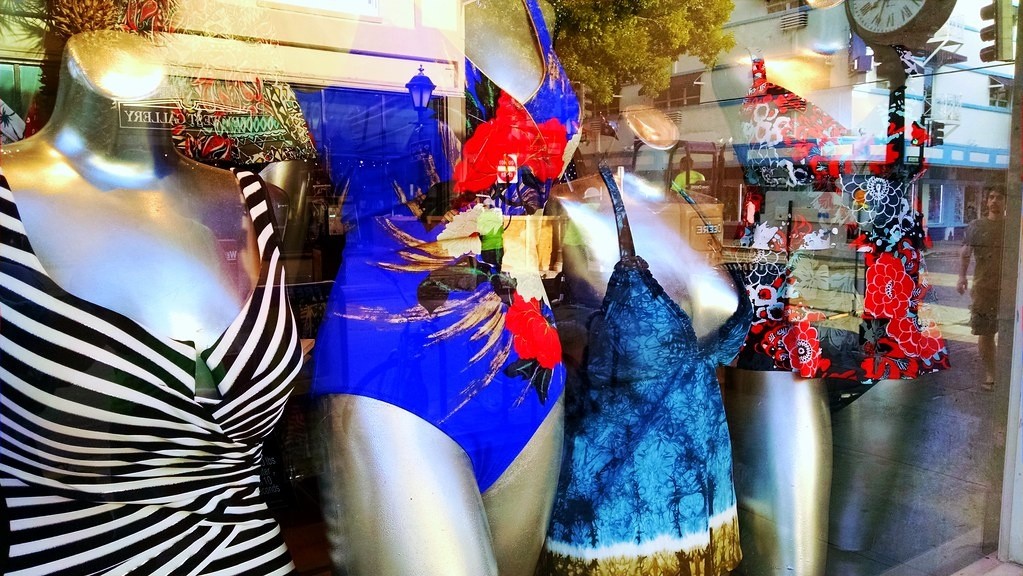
left=956, top=184, right=1007, bottom=391
left=302, top=0, right=582, bottom=576
left=671, top=157, right=705, bottom=193
left=0, top=28, right=303, bottom=576
left=746, top=199, right=755, bottom=246
left=561, top=188, right=601, bottom=304
left=476, top=197, right=503, bottom=292
left=710, top=0, right=949, bottom=576
left=546, top=105, right=754, bottom=576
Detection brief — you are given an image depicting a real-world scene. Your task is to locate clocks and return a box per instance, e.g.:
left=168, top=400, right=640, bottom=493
left=844, top=0, right=958, bottom=53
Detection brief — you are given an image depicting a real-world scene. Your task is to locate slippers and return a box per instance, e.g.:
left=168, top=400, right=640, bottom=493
left=982, top=380, right=994, bottom=391
